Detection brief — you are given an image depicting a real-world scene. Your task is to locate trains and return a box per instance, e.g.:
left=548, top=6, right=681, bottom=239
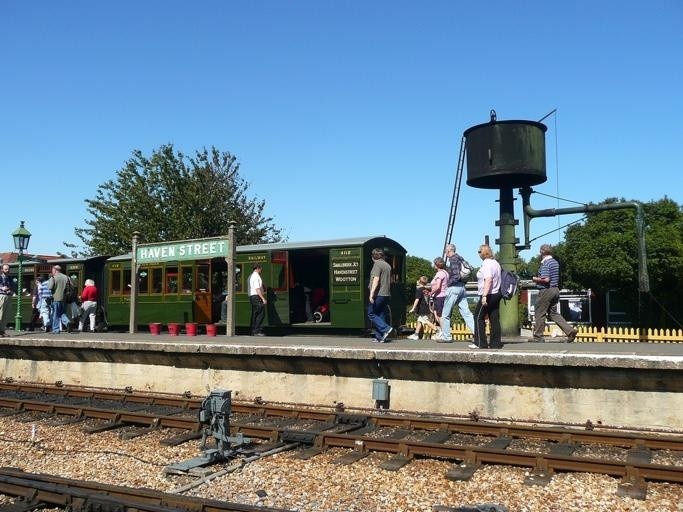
left=0, top=235, right=408, bottom=334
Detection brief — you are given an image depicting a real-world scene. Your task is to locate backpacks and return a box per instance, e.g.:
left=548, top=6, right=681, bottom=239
left=502, top=269, right=517, bottom=300
left=63, top=281, right=74, bottom=303
left=460, top=261, right=472, bottom=282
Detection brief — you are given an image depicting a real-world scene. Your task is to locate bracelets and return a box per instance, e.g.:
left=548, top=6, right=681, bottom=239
left=481, top=295, right=487, bottom=297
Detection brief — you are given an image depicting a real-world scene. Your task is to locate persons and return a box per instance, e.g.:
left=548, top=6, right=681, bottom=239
left=246, top=264, right=268, bottom=336
left=434, top=244, right=474, bottom=342
left=24, top=265, right=98, bottom=335
left=0, top=264, right=17, bottom=338
left=415, top=256, right=449, bottom=326
left=406, top=276, right=440, bottom=341
left=526, top=244, right=579, bottom=344
left=466, top=244, right=501, bottom=349
left=366, top=247, right=393, bottom=345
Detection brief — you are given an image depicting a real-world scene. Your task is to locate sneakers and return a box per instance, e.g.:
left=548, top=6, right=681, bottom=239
left=431, top=332, right=441, bottom=340
left=437, top=338, right=453, bottom=343
left=468, top=343, right=480, bottom=349
left=566, top=329, right=579, bottom=343
left=406, top=334, right=420, bottom=341
left=0, top=322, right=97, bottom=337
left=249, top=330, right=265, bottom=336
left=382, top=326, right=393, bottom=340
left=528, top=336, right=545, bottom=343
left=371, top=338, right=385, bottom=343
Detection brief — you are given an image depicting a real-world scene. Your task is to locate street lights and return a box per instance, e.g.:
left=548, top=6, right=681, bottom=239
left=11, top=220, right=31, bottom=332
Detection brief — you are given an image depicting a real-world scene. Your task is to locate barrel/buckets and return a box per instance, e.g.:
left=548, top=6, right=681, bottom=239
left=184, top=322, right=198, bottom=336
left=148, top=322, right=163, bottom=336
left=167, top=322, right=182, bottom=336
left=205, top=323, right=218, bottom=338
